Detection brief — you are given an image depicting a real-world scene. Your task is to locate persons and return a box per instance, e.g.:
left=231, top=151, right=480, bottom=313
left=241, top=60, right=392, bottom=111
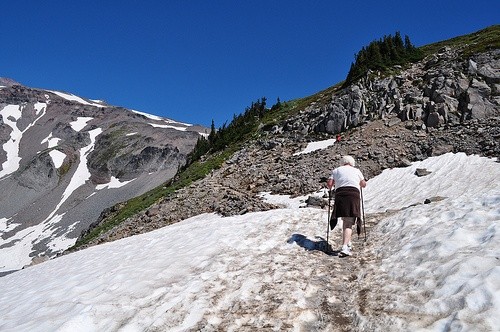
left=324, top=151, right=366, bottom=259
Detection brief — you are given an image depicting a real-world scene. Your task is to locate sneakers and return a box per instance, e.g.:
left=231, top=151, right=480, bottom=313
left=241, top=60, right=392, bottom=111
left=340, top=248, right=352, bottom=256
left=348, top=244, right=352, bottom=250
